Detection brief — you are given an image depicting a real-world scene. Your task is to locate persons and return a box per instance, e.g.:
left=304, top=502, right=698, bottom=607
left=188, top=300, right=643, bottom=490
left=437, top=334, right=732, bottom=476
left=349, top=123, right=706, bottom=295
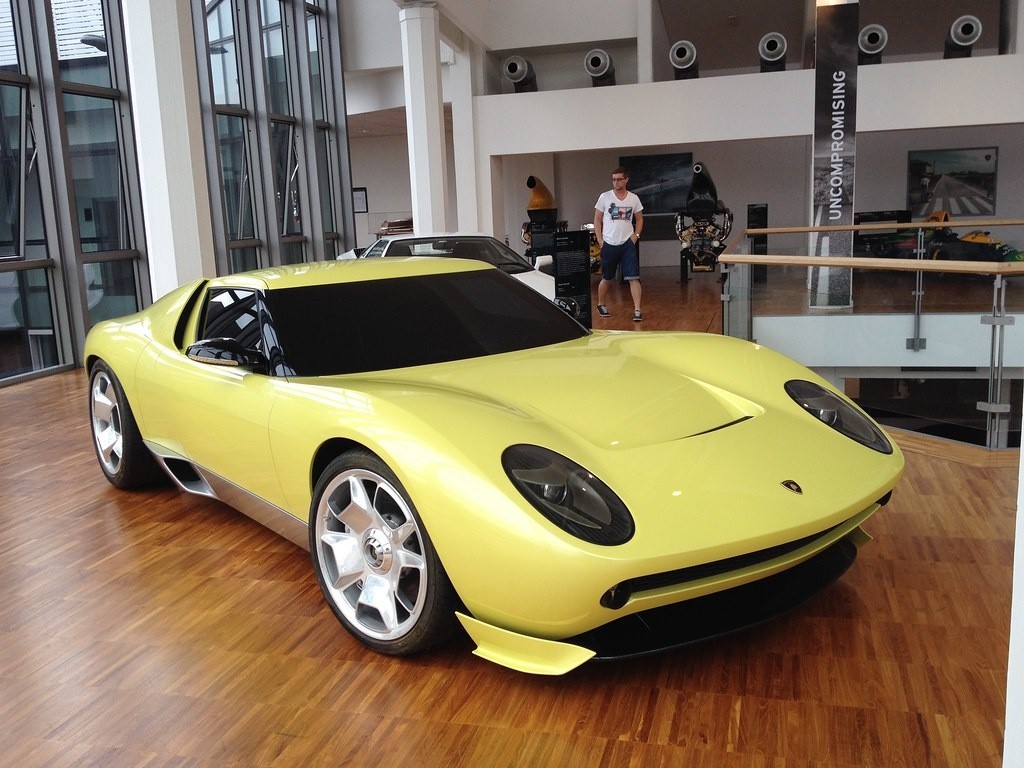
left=594, top=168, right=644, bottom=321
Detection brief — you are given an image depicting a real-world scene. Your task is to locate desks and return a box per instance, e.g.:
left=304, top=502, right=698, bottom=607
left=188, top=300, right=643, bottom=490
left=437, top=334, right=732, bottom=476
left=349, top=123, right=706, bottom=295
left=368, top=231, right=414, bottom=241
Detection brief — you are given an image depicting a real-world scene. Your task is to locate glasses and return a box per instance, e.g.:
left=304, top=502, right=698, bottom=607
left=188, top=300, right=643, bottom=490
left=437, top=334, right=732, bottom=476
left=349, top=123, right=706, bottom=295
left=612, top=177, right=626, bottom=182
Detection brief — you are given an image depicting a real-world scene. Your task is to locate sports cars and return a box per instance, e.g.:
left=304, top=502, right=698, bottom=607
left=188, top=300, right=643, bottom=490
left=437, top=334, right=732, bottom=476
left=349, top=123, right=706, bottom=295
left=84, top=256, right=905, bottom=677
left=334, top=232, right=555, bottom=303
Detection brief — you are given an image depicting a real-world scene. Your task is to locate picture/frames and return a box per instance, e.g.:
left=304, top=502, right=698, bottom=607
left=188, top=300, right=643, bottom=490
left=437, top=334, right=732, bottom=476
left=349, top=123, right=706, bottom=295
left=905, top=147, right=999, bottom=219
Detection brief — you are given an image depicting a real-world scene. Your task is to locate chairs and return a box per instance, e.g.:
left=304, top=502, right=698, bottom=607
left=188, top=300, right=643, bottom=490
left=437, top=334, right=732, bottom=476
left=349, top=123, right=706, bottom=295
left=520, top=222, right=532, bottom=264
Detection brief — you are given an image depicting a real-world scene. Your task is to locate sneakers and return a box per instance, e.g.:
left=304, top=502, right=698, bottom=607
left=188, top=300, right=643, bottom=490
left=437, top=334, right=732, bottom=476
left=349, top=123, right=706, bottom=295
left=596, top=304, right=611, bottom=317
left=633, top=310, right=643, bottom=321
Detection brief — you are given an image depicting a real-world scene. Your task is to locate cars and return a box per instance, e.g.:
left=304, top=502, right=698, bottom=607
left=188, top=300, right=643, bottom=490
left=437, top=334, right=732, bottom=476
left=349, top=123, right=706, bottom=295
left=873, top=210, right=1024, bottom=279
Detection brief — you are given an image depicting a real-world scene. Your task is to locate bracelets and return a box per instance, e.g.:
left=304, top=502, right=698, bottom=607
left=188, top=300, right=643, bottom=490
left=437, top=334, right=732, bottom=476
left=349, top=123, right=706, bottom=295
left=635, top=233, right=640, bottom=238
